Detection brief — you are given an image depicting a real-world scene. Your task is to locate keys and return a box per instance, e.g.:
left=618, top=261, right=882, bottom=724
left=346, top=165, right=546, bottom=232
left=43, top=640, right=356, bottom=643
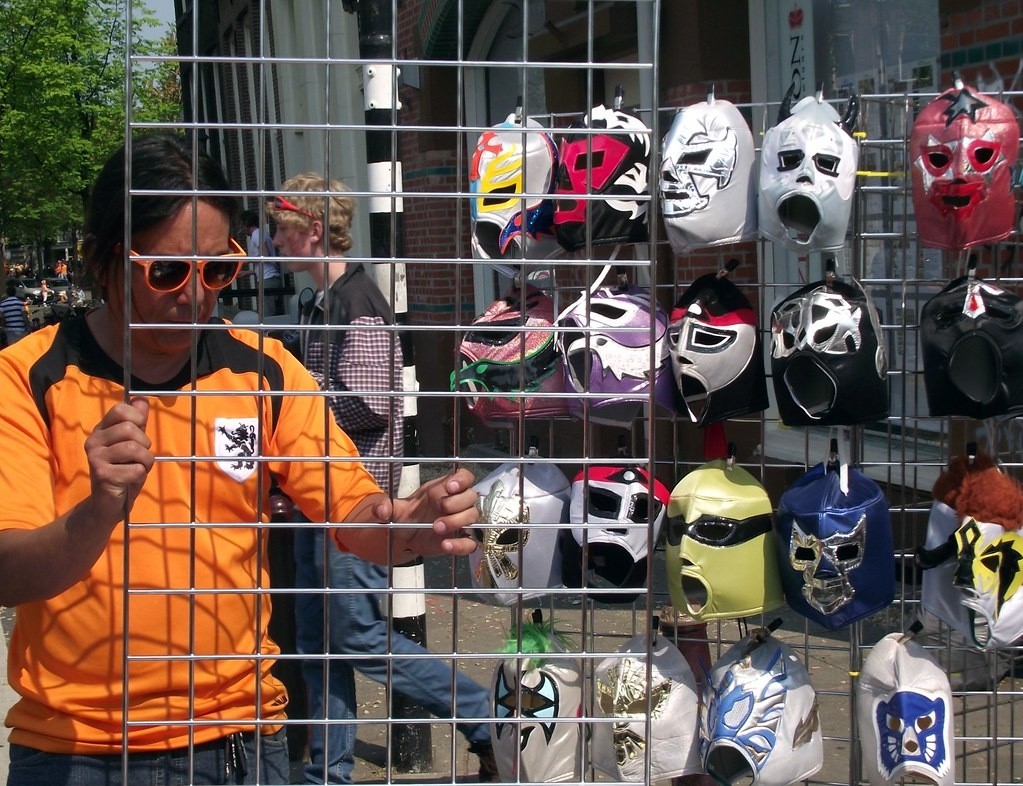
left=224, top=732, right=251, bottom=781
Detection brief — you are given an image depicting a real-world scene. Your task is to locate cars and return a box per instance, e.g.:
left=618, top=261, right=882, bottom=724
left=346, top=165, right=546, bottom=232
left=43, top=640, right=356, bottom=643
left=5, top=278, right=54, bottom=304
left=38, top=277, right=77, bottom=301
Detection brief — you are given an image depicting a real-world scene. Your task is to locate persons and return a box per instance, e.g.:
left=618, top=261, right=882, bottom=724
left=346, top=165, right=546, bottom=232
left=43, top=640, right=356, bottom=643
left=41, top=280, right=47, bottom=307
left=55, top=258, right=69, bottom=281
left=0, top=286, right=30, bottom=346
left=0, top=131, right=481, bottom=786
left=264, top=171, right=492, bottom=785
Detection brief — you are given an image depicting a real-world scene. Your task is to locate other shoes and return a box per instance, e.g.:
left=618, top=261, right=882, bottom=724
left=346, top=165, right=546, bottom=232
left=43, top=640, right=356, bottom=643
left=468, top=740, right=499, bottom=783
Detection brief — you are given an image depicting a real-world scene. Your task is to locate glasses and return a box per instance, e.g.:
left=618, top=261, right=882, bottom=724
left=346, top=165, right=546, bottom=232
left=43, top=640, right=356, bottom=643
left=114, top=235, right=248, bottom=293
left=275, top=195, right=318, bottom=220
